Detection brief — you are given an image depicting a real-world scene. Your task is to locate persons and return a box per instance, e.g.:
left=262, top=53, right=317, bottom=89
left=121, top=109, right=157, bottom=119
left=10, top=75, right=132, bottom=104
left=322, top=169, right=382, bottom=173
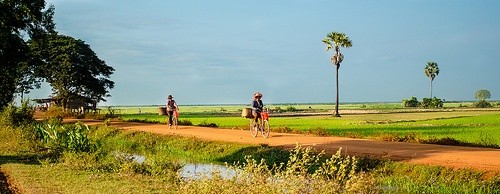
left=139, top=108, right=141, bottom=114
left=165, top=94, right=179, bottom=126
left=251, top=92, right=266, bottom=132
left=77, top=107, right=82, bottom=114
left=36, top=102, right=48, bottom=111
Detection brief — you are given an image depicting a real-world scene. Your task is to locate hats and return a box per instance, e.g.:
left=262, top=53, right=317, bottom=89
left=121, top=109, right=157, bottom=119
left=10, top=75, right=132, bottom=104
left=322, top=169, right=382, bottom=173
left=255, top=93, right=261, bottom=97
left=167, top=95, right=173, bottom=99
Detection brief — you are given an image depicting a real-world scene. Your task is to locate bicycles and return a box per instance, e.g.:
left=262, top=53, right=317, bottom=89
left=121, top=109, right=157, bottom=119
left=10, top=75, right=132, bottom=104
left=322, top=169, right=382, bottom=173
left=167, top=107, right=178, bottom=130
left=250, top=107, right=270, bottom=138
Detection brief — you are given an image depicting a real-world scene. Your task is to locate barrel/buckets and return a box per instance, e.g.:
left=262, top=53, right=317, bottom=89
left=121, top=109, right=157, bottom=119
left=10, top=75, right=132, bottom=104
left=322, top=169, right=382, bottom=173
left=159, top=107, right=167, bottom=116
left=242, top=108, right=252, bottom=118
